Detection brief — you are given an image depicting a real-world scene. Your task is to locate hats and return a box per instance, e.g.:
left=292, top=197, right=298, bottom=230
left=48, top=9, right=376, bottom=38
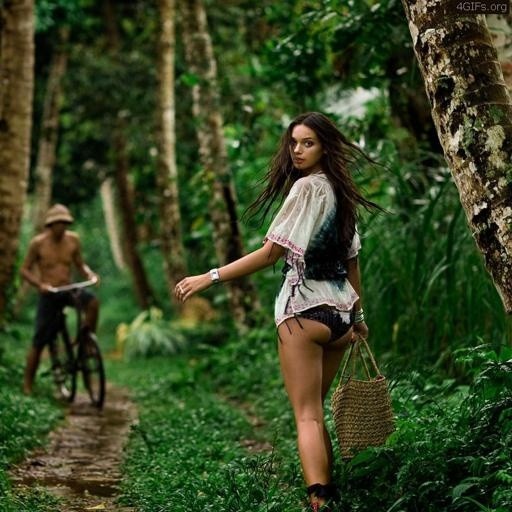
left=42, top=202, right=75, bottom=226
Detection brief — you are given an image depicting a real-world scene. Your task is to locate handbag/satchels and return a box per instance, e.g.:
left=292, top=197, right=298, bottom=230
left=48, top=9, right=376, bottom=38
left=330, top=331, right=398, bottom=463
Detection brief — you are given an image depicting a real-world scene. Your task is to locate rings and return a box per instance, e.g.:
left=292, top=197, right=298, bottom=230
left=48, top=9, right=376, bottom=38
left=179, top=285, right=182, bottom=290
left=181, top=290, right=185, bottom=294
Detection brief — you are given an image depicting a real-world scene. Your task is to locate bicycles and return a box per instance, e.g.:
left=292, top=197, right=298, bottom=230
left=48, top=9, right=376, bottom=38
left=40, top=279, right=104, bottom=408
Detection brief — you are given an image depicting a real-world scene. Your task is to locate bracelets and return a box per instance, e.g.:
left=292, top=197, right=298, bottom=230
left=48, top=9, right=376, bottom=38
left=210, top=269, right=220, bottom=284
left=355, top=307, right=364, bottom=323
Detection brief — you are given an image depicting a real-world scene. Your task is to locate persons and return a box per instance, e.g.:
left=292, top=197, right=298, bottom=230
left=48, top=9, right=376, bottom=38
left=18, top=204, right=101, bottom=395
left=173, top=110, right=398, bottom=512
